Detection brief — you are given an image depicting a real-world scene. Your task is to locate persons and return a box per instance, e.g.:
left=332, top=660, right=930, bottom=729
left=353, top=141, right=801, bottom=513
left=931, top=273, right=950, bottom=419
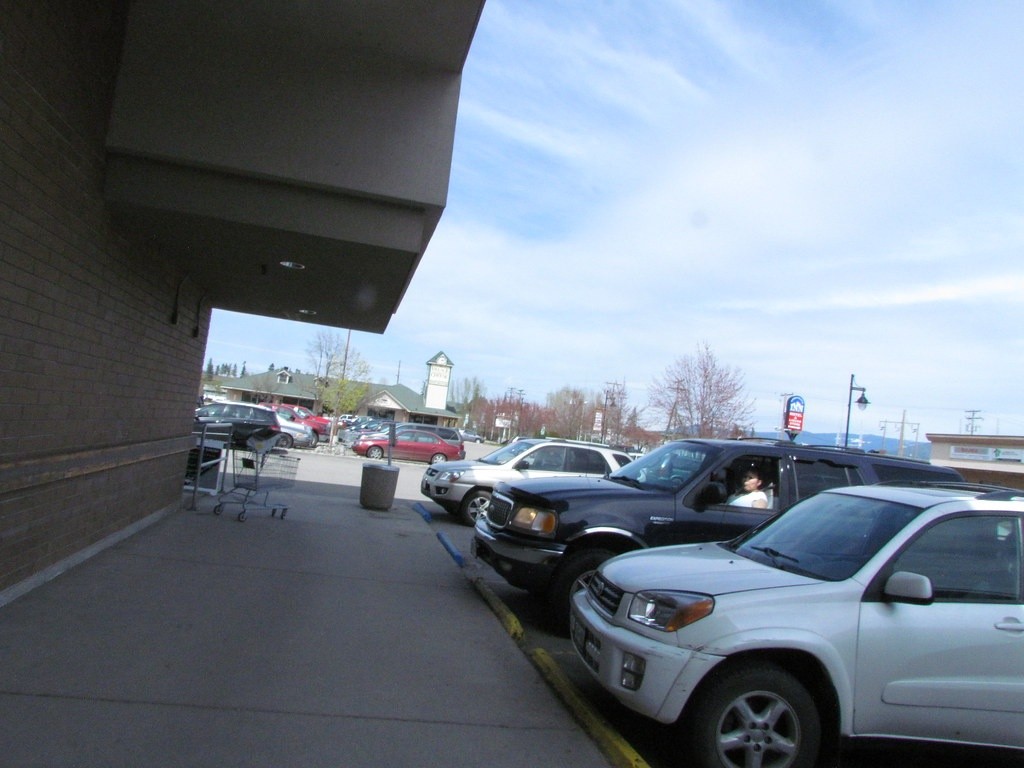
left=726, top=465, right=767, bottom=508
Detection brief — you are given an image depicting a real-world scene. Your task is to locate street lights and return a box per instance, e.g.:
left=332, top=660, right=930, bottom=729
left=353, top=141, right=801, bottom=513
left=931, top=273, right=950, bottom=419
left=600, top=390, right=618, bottom=444
left=845, top=374, right=871, bottom=449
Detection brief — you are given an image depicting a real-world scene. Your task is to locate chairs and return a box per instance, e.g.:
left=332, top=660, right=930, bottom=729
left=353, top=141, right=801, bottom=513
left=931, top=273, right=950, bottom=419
left=759, top=467, right=775, bottom=509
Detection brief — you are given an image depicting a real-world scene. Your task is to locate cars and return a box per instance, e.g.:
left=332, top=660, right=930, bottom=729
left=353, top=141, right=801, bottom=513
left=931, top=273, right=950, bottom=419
left=507, top=436, right=702, bottom=490
left=202, top=392, right=463, bottom=451
left=351, top=428, right=467, bottom=467
left=457, top=427, right=487, bottom=444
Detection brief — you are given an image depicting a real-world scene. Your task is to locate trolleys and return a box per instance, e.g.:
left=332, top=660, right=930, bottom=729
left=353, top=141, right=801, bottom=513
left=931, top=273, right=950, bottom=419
left=214, top=442, right=302, bottom=522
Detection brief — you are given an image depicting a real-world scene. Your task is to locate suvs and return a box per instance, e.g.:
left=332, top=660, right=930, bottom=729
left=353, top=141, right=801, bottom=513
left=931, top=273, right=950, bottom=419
left=568, top=480, right=1024, bottom=768
left=193, top=401, right=282, bottom=450
left=473, top=436, right=968, bottom=641
left=419, top=436, right=650, bottom=528
